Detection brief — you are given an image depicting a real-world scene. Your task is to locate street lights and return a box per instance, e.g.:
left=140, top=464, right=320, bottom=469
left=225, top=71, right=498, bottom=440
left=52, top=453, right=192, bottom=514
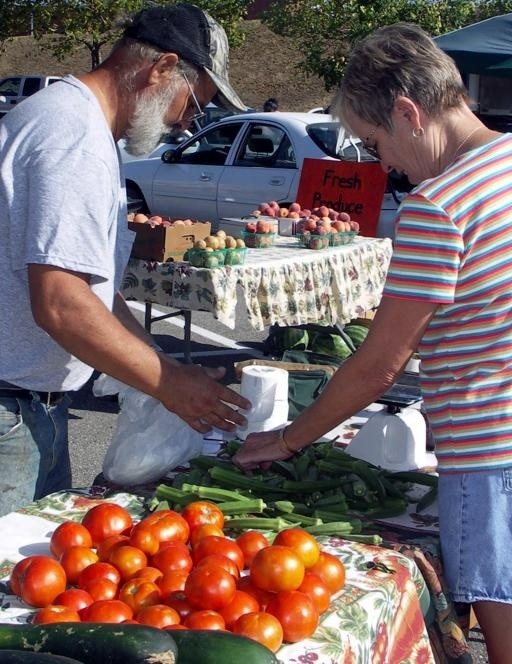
left=289, top=368, right=331, bottom=421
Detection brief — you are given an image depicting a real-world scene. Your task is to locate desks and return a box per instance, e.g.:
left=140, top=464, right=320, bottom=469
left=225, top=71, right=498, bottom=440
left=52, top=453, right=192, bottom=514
left=0, top=376, right=478, bottom=664
left=103, top=235, right=393, bottom=371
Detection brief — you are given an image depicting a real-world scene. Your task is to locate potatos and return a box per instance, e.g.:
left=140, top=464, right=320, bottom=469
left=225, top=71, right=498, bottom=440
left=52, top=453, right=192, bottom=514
left=0, top=622, right=278, bottom=664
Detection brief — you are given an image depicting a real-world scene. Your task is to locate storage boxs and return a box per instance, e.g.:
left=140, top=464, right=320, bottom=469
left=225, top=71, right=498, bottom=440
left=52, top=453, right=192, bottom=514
left=125, top=219, right=212, bottom=264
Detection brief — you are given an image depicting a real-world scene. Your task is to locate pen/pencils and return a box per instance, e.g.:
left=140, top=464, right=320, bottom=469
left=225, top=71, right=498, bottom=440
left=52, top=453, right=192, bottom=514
left=1, top=74, right=65, bottom=117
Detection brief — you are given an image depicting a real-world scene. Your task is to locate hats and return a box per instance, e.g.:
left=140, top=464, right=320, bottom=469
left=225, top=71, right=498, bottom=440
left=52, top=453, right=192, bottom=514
left=114, top=101, right=288, bottom=164
left=118, top=111, right=415, bottom=249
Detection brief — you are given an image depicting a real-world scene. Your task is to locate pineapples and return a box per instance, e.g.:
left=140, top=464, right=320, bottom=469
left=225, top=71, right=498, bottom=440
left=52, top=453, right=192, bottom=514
left=122, top=201, right=359, bottom=270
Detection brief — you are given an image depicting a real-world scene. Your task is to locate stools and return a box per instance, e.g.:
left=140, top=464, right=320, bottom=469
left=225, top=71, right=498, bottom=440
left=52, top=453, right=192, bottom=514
left=0, top=389, right=66, bottom=403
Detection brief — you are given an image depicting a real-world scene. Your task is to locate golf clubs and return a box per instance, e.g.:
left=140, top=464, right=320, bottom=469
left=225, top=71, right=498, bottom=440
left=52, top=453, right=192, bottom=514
left=273, top=321, right=373, bottom=361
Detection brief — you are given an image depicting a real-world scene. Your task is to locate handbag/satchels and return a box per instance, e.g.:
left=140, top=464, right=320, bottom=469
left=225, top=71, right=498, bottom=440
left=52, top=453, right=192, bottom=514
left=279, top=426, right=303, bottom=456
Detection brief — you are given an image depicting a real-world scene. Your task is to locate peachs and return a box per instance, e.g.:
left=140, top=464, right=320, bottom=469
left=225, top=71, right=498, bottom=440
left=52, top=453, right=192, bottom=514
left=122, top=201, right=359, bottom=270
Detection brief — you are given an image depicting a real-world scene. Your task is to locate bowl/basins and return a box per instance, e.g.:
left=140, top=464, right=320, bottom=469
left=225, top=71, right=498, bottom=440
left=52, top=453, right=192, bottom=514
left=452, top=125, right=485, bottom=158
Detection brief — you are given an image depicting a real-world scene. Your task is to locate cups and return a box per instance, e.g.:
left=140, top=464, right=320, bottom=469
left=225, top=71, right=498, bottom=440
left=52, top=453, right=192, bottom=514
left=183, top=73, right=207, bottom=122
left=360, top=123, right=382, bottom=160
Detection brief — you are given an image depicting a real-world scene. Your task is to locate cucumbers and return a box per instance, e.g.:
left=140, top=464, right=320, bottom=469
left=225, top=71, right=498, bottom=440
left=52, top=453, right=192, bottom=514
left=0, top=622, right=278, bottom=664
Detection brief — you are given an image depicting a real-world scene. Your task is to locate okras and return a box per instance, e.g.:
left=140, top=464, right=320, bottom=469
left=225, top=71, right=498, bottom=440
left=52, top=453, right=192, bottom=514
left=156, top=434, right=441, bottom=548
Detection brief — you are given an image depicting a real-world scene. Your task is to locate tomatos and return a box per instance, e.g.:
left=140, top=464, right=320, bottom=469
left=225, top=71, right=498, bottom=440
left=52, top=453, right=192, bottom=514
left=8, top=502, right=347, bottom=652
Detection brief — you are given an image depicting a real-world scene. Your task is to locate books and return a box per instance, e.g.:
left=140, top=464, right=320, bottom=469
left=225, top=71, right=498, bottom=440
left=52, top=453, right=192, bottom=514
left=125, top=219, right=212, bottom=264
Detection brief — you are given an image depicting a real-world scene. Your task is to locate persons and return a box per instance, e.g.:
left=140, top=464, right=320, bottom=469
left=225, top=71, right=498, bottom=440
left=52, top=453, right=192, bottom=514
left=230, top=21, right=512, bottom=663
left=263, top=98, right=280, bottom=112
left=0, top=4, right=252, bottom=524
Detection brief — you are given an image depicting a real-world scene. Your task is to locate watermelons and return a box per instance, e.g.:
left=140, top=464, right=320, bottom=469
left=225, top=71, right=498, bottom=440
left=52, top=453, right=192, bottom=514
left=273, top=321, right=373, bottom=361
left=344, top=371, right=438, bottom=475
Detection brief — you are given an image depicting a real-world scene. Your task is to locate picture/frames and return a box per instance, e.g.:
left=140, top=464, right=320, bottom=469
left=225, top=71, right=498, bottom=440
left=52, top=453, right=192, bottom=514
left=103, top=235, right=393, bottom=371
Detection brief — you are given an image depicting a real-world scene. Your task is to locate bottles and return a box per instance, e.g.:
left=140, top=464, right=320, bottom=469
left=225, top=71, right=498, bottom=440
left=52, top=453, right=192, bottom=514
left=124, top=4, right=248, bottom=114
